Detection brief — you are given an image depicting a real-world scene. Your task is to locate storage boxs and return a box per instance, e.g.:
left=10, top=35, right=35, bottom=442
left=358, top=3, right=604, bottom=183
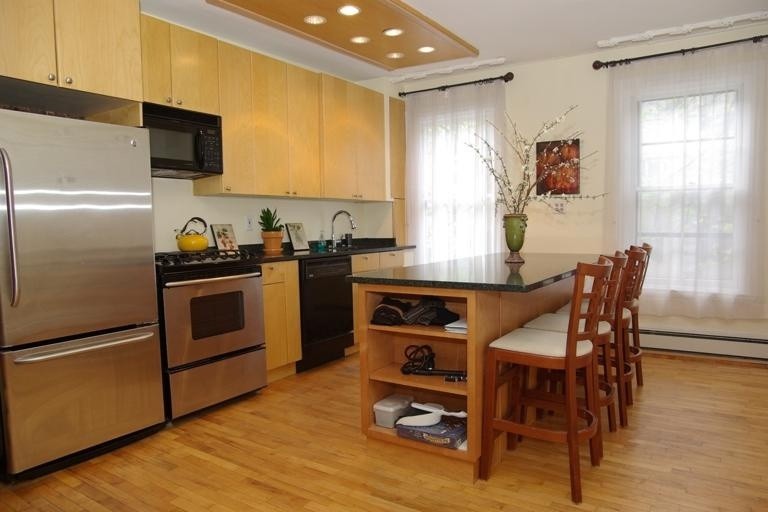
left=372, top=393, right=414, bottom=429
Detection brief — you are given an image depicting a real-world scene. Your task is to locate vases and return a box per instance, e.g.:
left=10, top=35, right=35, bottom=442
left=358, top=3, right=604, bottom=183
left=504, top=214, right=528, bottom=263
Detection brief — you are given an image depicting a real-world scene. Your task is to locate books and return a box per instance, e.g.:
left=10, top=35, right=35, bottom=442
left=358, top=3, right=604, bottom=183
left=444, top=318, right=468, bottom=334
left=210, top=224, right=241, bottom=255
left=285, top=223, right=310, bottom=251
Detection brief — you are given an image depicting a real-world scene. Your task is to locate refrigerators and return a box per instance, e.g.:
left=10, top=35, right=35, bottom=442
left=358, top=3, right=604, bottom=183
left=1, top=105, right=167, bottom=485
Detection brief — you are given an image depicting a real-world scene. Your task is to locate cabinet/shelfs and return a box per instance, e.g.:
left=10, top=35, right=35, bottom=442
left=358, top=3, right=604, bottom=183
left=140, top=11, right=220, bottom=117
left=193, top=38, right=321, bottom=200
left=0, top=0, right=143, bottom=101
left=320, top=72, right=386, bottom=203
left=358, top=283, right=500, bottom=462
left=351, top=249, right=406, bottom=343
left=257, top=260, right=303, bottom=372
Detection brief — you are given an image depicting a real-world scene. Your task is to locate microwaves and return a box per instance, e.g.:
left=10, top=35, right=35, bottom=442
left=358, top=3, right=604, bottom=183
left=138, top=104, right=223, bottom=180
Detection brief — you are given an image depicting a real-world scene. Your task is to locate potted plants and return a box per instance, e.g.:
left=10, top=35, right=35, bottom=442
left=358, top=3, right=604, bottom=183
left=258, top=206, right=285, bottom=256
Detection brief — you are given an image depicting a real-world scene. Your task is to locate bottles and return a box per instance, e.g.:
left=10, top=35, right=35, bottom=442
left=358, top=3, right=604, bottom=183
left=339, top=232, right=346, bottom=247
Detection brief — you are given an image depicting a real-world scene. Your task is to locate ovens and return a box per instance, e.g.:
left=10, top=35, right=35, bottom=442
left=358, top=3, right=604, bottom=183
left=162, top=271, right=266, bottom=421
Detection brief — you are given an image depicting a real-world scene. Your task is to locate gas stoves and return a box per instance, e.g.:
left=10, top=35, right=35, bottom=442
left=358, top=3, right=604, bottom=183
left=151, top=250, right=261, bottom=281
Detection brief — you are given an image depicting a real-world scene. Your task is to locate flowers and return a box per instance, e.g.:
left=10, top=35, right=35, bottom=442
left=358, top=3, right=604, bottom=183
left=457, top=101, right=609, bottom=216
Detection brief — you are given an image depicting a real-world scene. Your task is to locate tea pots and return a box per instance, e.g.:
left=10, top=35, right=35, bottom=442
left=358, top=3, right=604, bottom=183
left=172, top=217, right=210, bottom=252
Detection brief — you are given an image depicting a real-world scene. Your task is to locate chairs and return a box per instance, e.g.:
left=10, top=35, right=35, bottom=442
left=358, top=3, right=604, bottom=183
left=548, top=245, right=647, bottom=425
left=480, top=256, right=613, bottom=504
left=553, top=244, right=652, bottom=404
left=517, top=250, right=628, bottom=462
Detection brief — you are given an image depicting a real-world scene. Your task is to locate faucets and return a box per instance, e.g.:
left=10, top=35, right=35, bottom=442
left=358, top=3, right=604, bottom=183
left=332, top=210, right=357, bottom=253
